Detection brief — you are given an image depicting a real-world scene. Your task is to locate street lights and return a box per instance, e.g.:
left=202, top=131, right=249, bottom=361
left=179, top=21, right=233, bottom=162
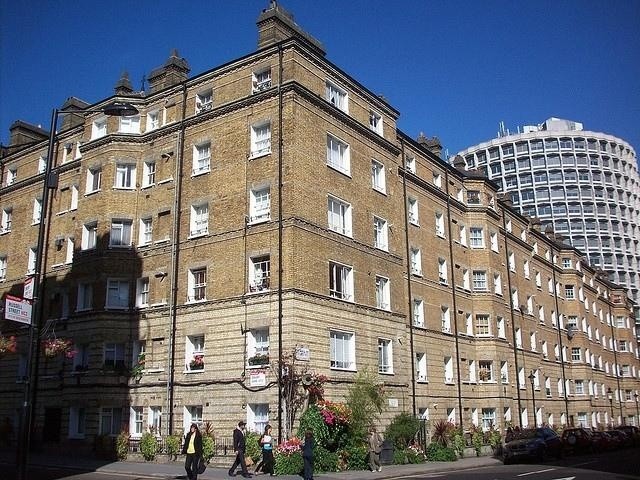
left=21, top=102, right=139, bottom=480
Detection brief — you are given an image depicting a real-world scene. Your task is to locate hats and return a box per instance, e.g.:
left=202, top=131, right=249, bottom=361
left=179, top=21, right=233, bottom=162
left=238, top=421, right=247, bottom=427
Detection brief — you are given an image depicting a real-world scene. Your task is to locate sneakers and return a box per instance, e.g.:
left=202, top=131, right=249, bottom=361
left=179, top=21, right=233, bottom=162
left=254, top=471, right=277, bottom=476
left=228, top=470, right=252, bottom=478
left=372, top=466, right=382, bottom=472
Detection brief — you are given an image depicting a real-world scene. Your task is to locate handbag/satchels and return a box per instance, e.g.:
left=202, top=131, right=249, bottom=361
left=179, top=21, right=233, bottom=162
left=196, top=462, right=206, bottom=474
left=375, top=446, right=383, bottom=454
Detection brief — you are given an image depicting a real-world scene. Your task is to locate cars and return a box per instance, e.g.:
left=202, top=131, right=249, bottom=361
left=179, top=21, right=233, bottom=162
left=502, top=426, right=640, bottom=465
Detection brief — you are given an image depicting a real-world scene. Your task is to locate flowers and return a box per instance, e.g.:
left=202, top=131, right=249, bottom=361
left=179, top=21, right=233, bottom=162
left=0, top=334, right=18, bottom=355
left=41, top=335, right=78, bottom=360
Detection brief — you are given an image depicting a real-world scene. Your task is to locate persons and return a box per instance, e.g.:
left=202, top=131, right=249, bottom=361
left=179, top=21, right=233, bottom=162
left=297, top=427, right=314, bottom=477
left=302, top=429, right=317, bottom=480
left=181, top=423, right=207, bottom=480
left=228, top=421, right=253, bottom=477
left=252, top=424, right=277, bottom=476
left=368, top=427, right=384, bottom=473
